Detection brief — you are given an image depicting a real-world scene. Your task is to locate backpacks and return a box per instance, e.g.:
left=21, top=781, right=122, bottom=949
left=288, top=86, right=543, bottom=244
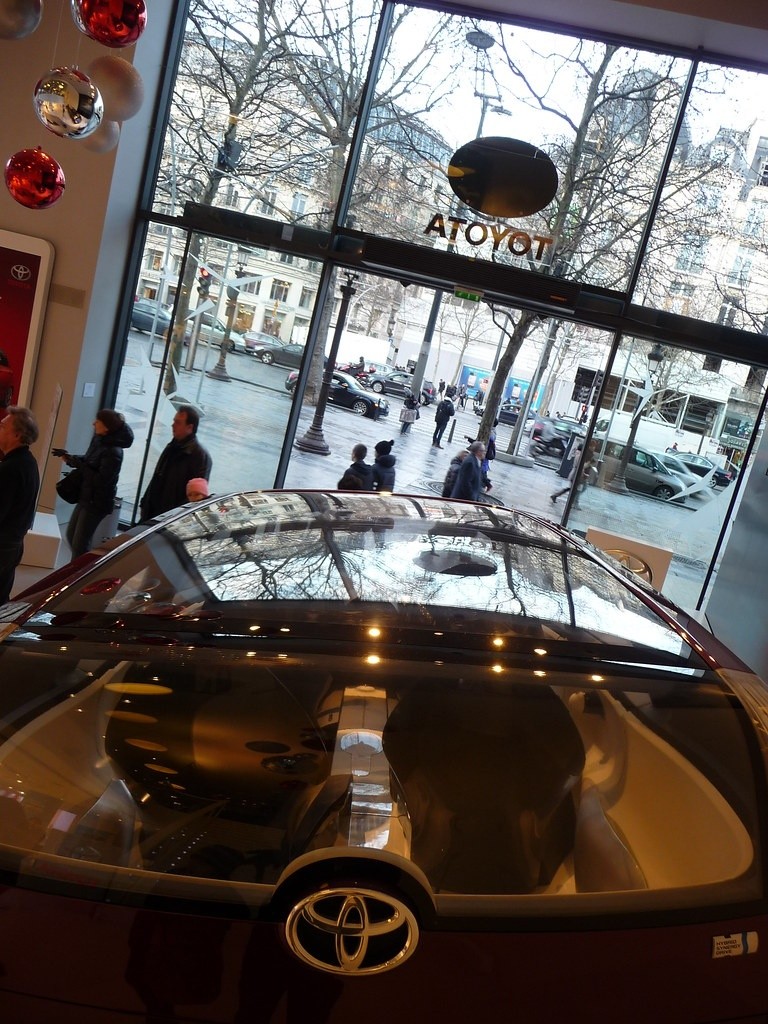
left=435, top=401, right=451, bottom=423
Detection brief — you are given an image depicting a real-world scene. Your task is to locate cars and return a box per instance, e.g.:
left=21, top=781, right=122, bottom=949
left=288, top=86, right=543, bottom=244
left=240, top=330, right=286, bottom=355
left=254, top=342, right=339, bottom=372
left=526, top=416, right=732, bottom=505
left=284, top=368, right=390, bottom=419
left=481, top=403, right=538, bottom=430
left=129, top=297, right=192, bottom=345
left=369, top=370, right=437, bottom=405
left=0, top=482, right=768, bottom=1024
left=185, top=308, right=246, bottom=354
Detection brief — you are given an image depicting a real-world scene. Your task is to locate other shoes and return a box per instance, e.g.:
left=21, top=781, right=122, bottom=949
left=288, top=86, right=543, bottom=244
left=436, top=445, right=444, bottom=449
left=486, top=485, right=492, bottom=492
left=551, top=495, right=557, bottom=503
left=432, top=444, right=435, bottom=445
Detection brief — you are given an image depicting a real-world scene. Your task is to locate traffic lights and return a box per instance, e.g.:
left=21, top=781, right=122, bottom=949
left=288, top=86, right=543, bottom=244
left=197, top=266, right=212, bottom=295
left=578, top=386, right=590, bottom=404
left=593, top=369, right=604, bottom=388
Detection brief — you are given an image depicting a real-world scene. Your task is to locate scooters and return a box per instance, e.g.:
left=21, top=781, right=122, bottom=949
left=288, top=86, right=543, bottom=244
left=337, top=361, right=377, bottom=388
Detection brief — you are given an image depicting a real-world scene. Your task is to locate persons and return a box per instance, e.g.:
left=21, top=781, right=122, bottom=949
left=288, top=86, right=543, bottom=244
left=350, top=356, right=364, bottom=376
left=550, top=440, right=596, bottom=510
left=545, top=410, right=549, bottom=417
left=0, top=406, right=40, bottom=608
left=504, top=397, right=520, bottom=411
left=51, top=409, right=134, bottom=563
left=400, top=387, right=420, bottom=436
left=672, top=442, right=678, bottom=450
left=186, top=478, right=208, bottom=502
left=556, top=412, right=561, bottom=419
left=441, top=418, right=499, bottom=502
left=138, top=406, right=212, bottom=527
left=438, top=379, right=468, bottom=405
left=395, top=365, right=407, bottom=378
left=432, top=389, right=455, bottom=449
left=473, top=390, right=485, bottom=412
left=338, top=440, right=396, bottom=492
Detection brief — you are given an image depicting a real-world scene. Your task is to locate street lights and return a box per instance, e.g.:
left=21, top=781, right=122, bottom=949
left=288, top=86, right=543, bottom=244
left=602, top=342, right=665, bottom=497
left=205, top=242, right=253, bottom=383
left=295, top=268, right=361, bottom=456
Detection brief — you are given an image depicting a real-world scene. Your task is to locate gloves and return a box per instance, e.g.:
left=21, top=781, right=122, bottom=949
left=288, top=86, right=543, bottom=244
left=51, top=448, right=69, bottom=457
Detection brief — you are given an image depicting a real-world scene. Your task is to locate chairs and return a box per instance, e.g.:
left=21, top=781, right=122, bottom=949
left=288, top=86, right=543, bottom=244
left=105, top=657, right=332, bottom=828
left=384, top=674, right=586, bottom=892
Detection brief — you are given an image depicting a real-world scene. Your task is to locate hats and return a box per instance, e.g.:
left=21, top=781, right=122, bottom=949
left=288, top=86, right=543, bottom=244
left=375, top=440, right=394, bottom=455
left=186, top=478, right=208, bottom=496
left=404, top=386, right=412, bottom=396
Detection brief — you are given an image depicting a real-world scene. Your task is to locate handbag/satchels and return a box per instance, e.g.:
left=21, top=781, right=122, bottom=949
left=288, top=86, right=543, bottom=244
left=486, top=444, right=496, bottom=460
left=399, top=408, right=420, bottom=423
left=56, top=470, right=83, bottom=504
